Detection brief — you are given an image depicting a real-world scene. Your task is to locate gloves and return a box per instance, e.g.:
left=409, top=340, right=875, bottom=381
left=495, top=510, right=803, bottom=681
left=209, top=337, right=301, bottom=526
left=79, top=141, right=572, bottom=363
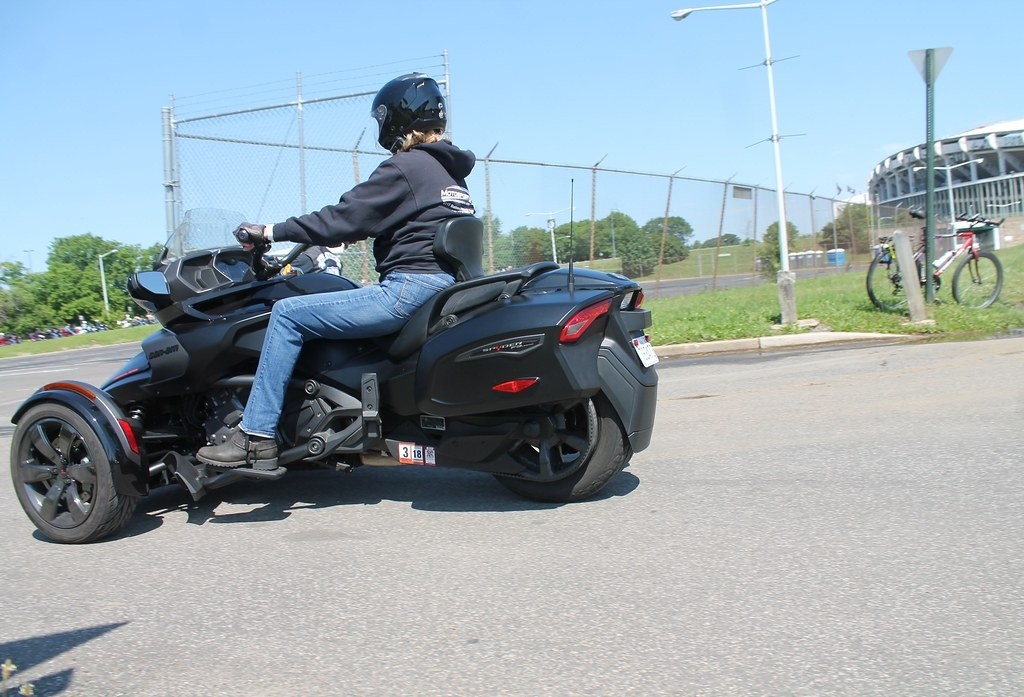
left=232, top=222, right=266, bottom=252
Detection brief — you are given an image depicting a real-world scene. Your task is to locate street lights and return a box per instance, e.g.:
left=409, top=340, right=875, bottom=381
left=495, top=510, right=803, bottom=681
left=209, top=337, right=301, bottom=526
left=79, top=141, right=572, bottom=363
left=97, top=247, right=118, bottom=326
left=554, top=236, right=571, bottom=263
left=526, top=206, right=575, bottom=264
left=609, top=207, right=621, bottom=256
left=668, top=0, right=795, bottom=321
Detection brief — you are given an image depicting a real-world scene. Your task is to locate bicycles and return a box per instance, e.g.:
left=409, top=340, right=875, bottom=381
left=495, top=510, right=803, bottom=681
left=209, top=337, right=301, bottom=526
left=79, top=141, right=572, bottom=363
left=866, top=206, right=1007, bottom=316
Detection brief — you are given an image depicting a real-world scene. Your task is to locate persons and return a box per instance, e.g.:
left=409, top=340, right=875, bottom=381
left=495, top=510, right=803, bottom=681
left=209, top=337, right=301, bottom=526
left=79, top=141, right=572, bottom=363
left=0, top=333, right=22, bottom=347
left=195, top=71, right=476, bottom=470
left=29, top=315, right=108, bottom=342
left=495, top=266, right=512, bottom=272
left=121, top=315, right=158, bottom=328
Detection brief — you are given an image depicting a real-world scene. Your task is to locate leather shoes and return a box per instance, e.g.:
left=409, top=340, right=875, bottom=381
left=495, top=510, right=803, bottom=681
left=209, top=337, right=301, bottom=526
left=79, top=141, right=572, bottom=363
left=196, top=427, right=279, bottom=470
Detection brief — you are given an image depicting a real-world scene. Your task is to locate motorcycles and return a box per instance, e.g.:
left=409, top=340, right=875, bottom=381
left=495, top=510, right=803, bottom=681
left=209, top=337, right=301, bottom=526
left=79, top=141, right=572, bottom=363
left=9, top=230, right=658, bottom=545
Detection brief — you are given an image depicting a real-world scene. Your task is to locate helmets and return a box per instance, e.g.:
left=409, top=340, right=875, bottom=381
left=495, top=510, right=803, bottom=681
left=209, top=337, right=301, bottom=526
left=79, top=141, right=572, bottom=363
left=371, top=72, right=447, bottom=153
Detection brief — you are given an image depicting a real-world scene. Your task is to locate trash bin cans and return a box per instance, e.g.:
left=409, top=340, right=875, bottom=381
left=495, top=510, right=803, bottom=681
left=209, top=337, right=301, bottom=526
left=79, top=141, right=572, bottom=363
left=872, top=243, right=892, bottom=263
left=814, top=250, right=823, bottom=267
left=827, top=248, right=846, bottom=266
left=797, top=252, right=806, bottom=268
left=805, top=250, right=814, bottom=266
left=788, top=253, right=797, bottom=268
